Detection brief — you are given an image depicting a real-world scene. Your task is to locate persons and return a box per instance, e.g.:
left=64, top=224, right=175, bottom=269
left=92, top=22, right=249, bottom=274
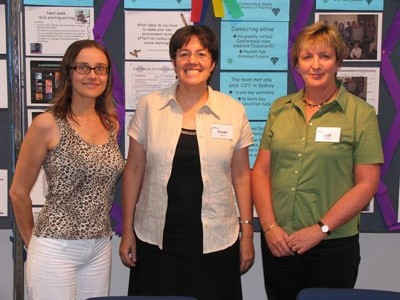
left=252, top=22, right=384, bottom=300
left=334, top=18, right=377, bottom=59
left=344, top=77, right=366, bottom=98
left=8, top=40, right=124, bottom=300
left=119, top=25, right=255, bottom=300
left=77, top=10, right=87, bottom=24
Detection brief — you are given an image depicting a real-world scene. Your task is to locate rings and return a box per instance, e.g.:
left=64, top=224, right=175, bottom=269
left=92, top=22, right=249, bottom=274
left=294, top=246, right=299, bottom=250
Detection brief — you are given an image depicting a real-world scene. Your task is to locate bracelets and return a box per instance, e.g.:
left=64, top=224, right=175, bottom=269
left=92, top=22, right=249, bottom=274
left=264, top=224, right=278, bottom=232
left=241, top=220, right=253, bottom=224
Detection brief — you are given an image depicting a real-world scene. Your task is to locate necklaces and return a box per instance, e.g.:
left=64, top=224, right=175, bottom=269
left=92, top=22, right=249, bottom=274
left=303, top=86, right=338, bottom=108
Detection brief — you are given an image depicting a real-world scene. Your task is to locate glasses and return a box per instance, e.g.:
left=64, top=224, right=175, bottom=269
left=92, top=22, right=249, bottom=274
left=68, top=63, right=109, bottom=75
left=178, top=50, right=211, bottom=61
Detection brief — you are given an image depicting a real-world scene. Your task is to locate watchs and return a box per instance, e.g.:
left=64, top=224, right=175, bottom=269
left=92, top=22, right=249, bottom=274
left=318, top=221, right=331, bottom=236
